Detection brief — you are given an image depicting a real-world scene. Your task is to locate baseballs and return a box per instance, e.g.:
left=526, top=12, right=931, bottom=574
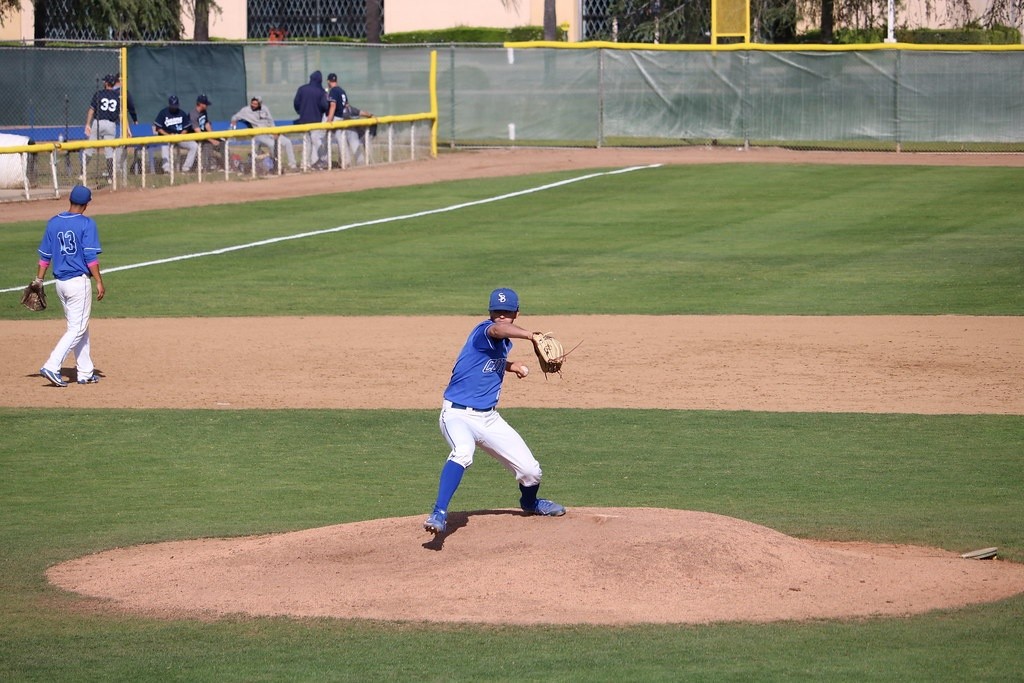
left=521, top=366, right=529, bottom=374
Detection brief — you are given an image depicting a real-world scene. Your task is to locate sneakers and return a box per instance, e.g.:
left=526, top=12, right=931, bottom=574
left=77, top=375, right=99, bottom=383
left=40, top=368, right=67, bottom=386
left=423, top=509, right=447, bottom=534
left=519, top=497, right=566, bottom=516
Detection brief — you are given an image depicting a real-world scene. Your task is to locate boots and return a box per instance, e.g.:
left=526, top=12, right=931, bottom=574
left=106, top=158, right=113, bottom=181
left=78, top=153, right=91, bottom=180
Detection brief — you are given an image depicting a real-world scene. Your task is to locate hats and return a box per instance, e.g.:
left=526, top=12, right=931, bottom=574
left=168, top=95, right=179, bottom=108
left=488, top=288, right=520, bottom=312
left=327, top=73, right=338, bottom=82
left=69, top=185, right=92, bottom=204
left=197, top=94, right=212, bottom=105
left=102, top=74, right=116, bottom=86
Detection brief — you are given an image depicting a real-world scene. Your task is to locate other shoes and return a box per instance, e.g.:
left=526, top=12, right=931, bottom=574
left=310, top=159, right=327, bottom=171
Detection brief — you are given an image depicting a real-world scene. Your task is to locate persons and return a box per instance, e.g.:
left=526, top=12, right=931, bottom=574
left=77, top=72, right=138, bottom=180
left=423, top=287, right=584, bottom=535
left=294, top=71, right=374, bottom=171
left=231, top=96, right=297, bottom=169
left=20, top=185, right=105, bottom=387
left=188, top=94, right=230, bottom=174
left=153, top=96, right=198, bottom=175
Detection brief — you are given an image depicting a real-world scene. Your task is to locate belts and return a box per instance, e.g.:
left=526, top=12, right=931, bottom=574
left=452, top=402, right=495, bottom=411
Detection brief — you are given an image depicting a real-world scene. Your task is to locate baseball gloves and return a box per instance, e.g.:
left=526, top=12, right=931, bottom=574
left=532, top=330, right=564, bottom=373
left=21, top=279, right=48, bottom=313
